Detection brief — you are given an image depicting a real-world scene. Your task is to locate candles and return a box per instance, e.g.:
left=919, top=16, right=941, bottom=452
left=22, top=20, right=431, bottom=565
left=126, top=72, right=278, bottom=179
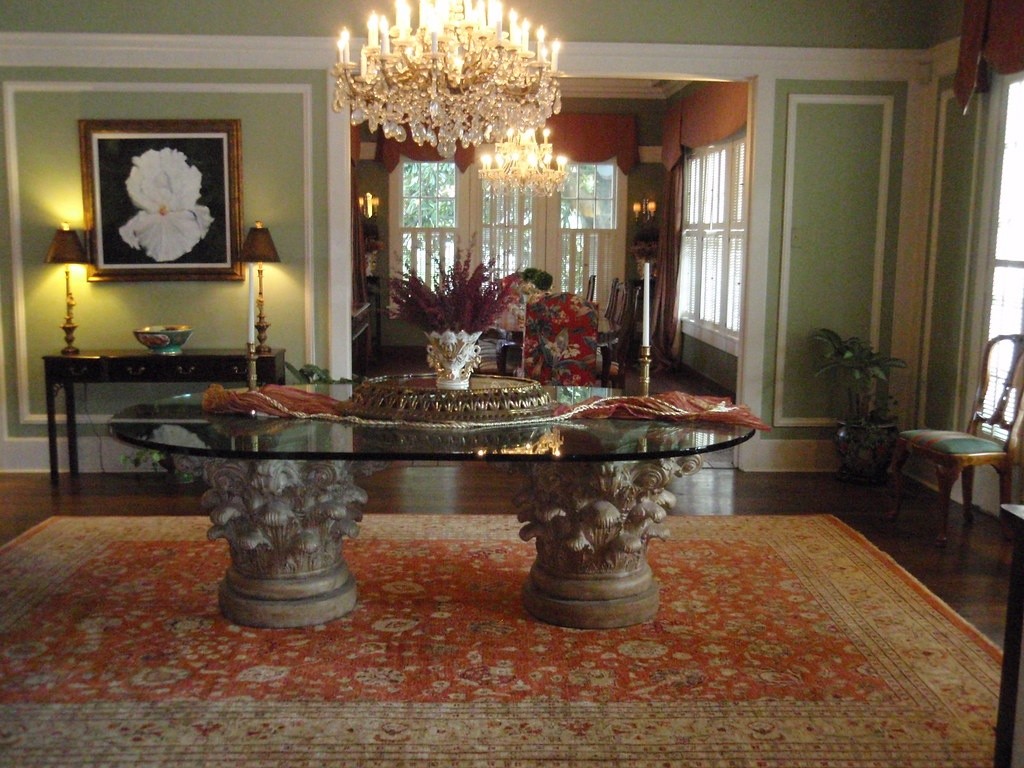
left=643, top=262, right=650, bottom=346
left=248, top=263, right=254, bottom=343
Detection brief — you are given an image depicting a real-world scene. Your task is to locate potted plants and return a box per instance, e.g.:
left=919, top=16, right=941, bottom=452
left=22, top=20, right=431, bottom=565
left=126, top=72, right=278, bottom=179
left=380, top=247, right=523, bottom=390
left=811, top=328, right=909, bottom=484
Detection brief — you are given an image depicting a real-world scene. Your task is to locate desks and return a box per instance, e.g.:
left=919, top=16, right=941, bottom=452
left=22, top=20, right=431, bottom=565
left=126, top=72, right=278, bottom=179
left=42, top=348, right=288, bottom=488
left=102, top=371, right=773, bottom=629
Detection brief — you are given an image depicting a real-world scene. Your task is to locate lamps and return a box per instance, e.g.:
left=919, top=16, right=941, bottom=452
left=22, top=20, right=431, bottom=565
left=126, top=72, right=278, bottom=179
left=239, top=220, right=282, bottom=353
left=326, top=0, right=569, bottom=159
left=42, top=222, right=92, bottom=354
left=478, top=128, right=570, bottom=198
left=632, top=198, right=657, bottom=227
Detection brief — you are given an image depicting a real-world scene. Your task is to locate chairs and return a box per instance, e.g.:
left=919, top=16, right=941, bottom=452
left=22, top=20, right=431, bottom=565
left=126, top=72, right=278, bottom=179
left=586, top=274, right=642, bottom=389
left=882, top=333, right=1024, bottom=550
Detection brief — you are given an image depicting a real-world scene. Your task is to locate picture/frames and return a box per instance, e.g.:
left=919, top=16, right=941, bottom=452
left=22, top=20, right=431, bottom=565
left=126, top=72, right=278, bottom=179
left=76, top=117, right=247, bottom=283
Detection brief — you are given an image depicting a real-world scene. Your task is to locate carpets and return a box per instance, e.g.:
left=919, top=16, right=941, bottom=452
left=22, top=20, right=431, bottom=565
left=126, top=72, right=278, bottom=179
left=0, top=511, right=1004, bottom=768
